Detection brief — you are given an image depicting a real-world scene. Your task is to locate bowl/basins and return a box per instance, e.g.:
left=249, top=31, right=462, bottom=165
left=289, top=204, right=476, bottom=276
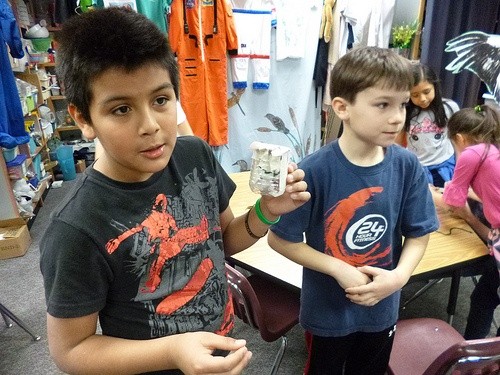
left=31, top=39, right=51, bottom=51
left=26, top=24, right=49, bottom=39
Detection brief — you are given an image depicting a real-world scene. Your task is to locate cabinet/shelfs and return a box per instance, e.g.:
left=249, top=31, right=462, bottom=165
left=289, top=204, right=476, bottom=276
left=0, top=61, right=94, bottom=227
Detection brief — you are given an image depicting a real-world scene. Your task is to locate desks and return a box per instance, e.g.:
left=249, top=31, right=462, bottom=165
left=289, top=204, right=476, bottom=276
left=223, top=169, right=490, bottom=302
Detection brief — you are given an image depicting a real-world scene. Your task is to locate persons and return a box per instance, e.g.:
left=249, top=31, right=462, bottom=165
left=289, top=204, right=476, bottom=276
left=443, top=104, right=500, bottom=341
left=402, top=60, right=462, bottom=187
left=38, top=5, right=311, bottom=375
left=267, top=46, right=440, bottom=375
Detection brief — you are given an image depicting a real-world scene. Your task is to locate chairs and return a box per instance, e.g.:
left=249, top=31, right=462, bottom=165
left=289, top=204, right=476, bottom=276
left=388, top=313, right=500, bottom=375
left=223, top=260, right=302, bottom=375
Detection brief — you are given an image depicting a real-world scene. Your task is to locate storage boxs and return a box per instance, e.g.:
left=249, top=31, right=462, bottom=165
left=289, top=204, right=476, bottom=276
left=7, top=155, right=29, bottom=179
left=41, top=169, right=46, bottom=177
left=40, top=162, right=45, bottom=171
left=3, top=144, right=19, bottom=161
left=0, top=214, right=33, bottom=260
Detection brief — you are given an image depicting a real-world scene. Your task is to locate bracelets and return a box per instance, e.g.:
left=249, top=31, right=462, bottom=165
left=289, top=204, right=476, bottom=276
left=255, top=197, right=280, bottom=225
left=245, top=205, right=269, bottom=239
